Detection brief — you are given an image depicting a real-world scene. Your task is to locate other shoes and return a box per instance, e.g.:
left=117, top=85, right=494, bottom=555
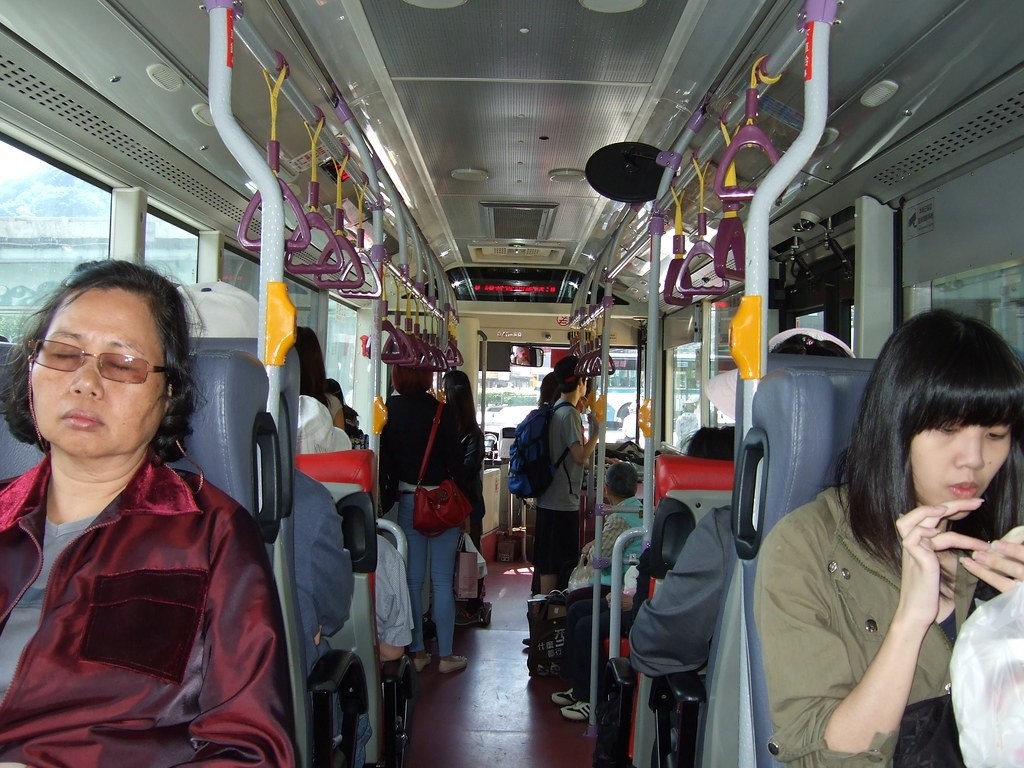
left=439, top=655, right=467, bottom=674
left=413, top=653, right=432, bottom=672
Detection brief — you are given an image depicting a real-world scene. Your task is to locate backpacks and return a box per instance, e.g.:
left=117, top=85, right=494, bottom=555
left=507, top=402, right=582, bottom=499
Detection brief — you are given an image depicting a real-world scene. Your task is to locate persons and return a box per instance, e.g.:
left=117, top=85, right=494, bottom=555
left=628, top=334, right=849, bottom=677
left=294, top=326, right=359, bottom=431
left=177, top=282, right=372, bottom=768
left=522, top=461, right=644, bottom=648
left=623, top=402, right=636, bottom=438
left=374, top=534, right=415, bottom=662
left=512, top=348, right=529, bottom=366
left=552, top=546, right=652, bottom=721
left=380, top=362, right=474, bottom=674
left=443, top=370, right=486, bottom=626
left=675, top=399, right=698, bottom=450
left=0, top=259, right=295, bottom=768
left=752, top=308, right=1024, bottom=768
left=529, top=355, right=599, bottom=597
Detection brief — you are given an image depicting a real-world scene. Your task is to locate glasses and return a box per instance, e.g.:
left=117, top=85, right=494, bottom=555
left=28, top=338, right=168, bottom=384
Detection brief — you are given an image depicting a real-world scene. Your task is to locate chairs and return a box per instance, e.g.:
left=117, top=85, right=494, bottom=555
left=626, top=353, right=878, bottom=767
left=0, top=285, right=414, bottom=768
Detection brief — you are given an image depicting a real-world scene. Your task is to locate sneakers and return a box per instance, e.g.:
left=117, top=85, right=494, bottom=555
left=480, top=601, right=492, bottom=624
left=560, top=701, right=589, bottom=722
left=454, top=607, right=482, bottom=625
left=552, top=688, right=578, bottom=705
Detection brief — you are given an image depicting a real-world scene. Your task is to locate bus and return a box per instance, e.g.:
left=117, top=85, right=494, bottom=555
left=583, top=352, right=738, bottom=431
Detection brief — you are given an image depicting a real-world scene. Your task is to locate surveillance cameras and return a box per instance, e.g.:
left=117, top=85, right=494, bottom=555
left=543, top=332, right=552, bottom=341
left=800, top=211, right=820, bottom=231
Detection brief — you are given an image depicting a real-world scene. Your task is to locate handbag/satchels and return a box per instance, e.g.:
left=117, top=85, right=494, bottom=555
left=414, top=481, right=472, bottom=537
left=892, top=692, right=965, bottom=768
left=567, top=553, right=593, bottom=594
left=454, top=533, right=487, bottom=599
left=949, top=579, right=1024, bottom=768
left=527, top=589, right=570, bottom=677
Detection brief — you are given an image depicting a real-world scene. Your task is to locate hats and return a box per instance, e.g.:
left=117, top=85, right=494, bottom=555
left=555, top=355, right=579, bottom=384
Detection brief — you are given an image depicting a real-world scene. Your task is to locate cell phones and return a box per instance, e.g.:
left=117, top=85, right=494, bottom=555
left=976, top=526, right=1024, bottom=570
